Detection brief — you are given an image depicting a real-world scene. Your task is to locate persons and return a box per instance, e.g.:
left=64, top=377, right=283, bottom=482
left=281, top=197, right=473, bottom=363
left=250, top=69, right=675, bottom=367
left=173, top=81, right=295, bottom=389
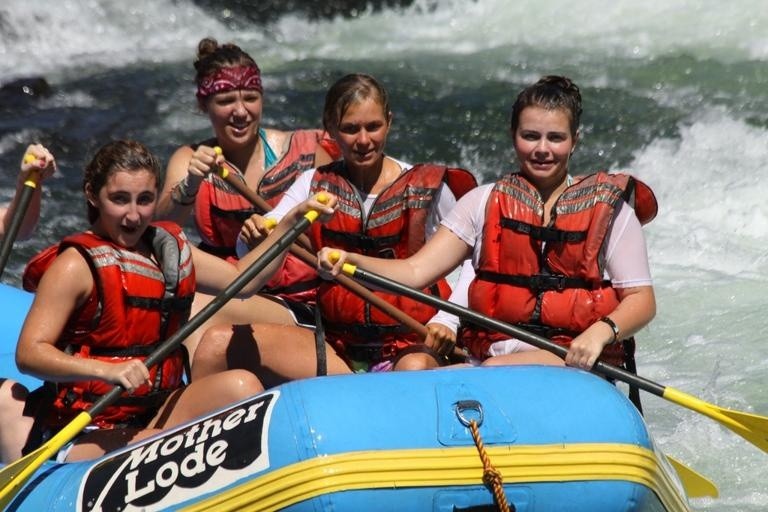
left=187, top=68, right=484, bottom=378
left=152, top=37, right=342, bottom=376
left=0, top=138, right=340, bottom=470
left=315, top=70, right=658, bottom=377
left=0, top=141, right=59, bottom=469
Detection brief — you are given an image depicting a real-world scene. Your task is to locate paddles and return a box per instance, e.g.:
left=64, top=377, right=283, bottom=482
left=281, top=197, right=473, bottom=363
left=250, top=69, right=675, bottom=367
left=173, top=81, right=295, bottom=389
left=0, top=192, right=328, bottom=512
left=330, top=251, right=768, bottom=498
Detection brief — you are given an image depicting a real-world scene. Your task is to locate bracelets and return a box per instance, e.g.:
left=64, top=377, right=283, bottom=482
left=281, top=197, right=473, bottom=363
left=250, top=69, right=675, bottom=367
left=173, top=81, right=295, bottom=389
left=178, top=177, right=199, bottom=197
left=182, top=173, right=196, bottom=189
left=169, top=184, right=196, bottom=207
left=597, top=314, right=621, bottom=349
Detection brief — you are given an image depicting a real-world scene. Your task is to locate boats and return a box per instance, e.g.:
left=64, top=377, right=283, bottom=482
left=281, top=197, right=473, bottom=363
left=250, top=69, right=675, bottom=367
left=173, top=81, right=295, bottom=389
left=0, top=283, right=692, bottom=512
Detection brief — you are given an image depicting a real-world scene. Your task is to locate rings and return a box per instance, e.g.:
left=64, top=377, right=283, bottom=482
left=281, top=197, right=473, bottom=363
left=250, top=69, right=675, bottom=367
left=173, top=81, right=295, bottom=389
left=249, top=226, right=257, bottom=233
